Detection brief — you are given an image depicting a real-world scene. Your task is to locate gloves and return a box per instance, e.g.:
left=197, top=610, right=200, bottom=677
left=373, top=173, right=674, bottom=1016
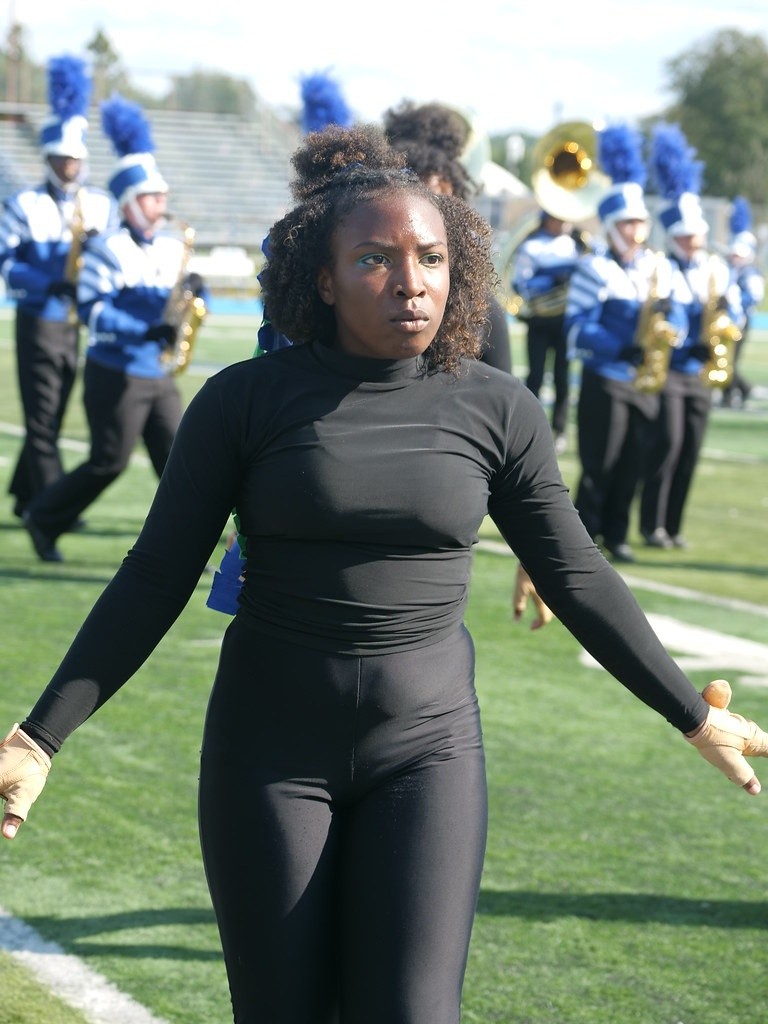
left=50, top=281, right=77, bottom=299
left=145, top=326, right=178, bottom=348
left=615, top=344, right=643, bottom=369
left=689, top=343, right=710, bottom=365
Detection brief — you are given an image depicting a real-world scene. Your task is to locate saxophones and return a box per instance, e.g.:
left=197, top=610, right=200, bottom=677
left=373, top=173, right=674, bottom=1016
left=691, top=250, right=745, bottom=393
left=67, top=179, right=89, bottom=330
left=633, top=235, right=685, bottom=394
left=161, top=210, right=208, bottom=377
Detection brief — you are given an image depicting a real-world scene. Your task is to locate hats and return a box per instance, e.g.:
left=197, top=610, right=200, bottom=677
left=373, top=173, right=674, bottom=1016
left=597, top=182, right=647, bottom=235
left=729, top=230, right=759, bottom=259
left=39, top=114, right=91, bottom=161
left=100, top=151, right=168, bottom=208
left=659, top=192, right=709, bottom=240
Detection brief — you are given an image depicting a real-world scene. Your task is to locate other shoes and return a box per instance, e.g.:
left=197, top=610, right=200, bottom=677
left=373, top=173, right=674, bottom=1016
left=604, top=539, right=634, bottom=564
left=549, top=428, right=568, bottom=457
left=641, top=520, right=687, bottom=550
left=23, top=513, right=62, bottom=561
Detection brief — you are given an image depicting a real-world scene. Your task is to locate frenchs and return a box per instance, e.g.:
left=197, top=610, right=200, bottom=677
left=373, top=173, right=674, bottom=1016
left=492, top=116, right=613, bottom=324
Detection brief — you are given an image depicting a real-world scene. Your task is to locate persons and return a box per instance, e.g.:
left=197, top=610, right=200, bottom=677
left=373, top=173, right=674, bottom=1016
left=2, top=55, right=767, bottom=631
left=1, top=121, right=767, bottom=1024
